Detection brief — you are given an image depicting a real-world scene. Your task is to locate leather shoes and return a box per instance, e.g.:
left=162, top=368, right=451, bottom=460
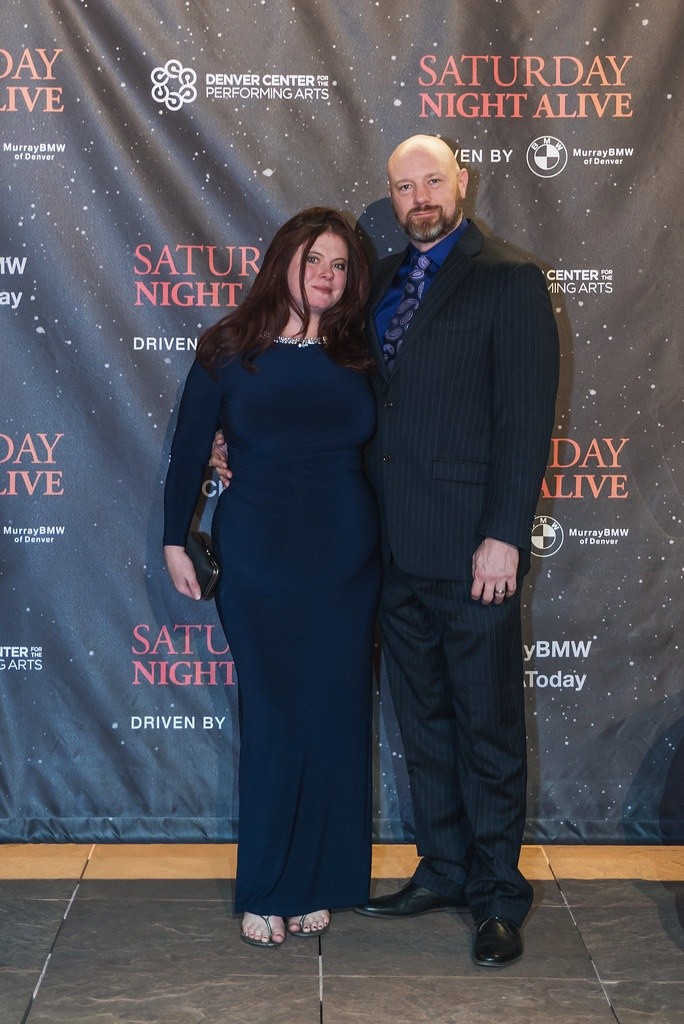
left=354, top=882, right=471, bottom=918
left=472, top=915, right=524, bottom=966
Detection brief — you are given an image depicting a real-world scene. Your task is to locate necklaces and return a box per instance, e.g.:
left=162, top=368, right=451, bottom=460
left=259, top=329, right=328, bottom=348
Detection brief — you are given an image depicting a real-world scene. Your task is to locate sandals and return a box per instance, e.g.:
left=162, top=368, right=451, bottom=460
left=286, top=908, right=332, bottom=938
left=240, top=911, right=286, bottom=946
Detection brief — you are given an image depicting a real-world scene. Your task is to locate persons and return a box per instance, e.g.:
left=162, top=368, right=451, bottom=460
left=163, top=207, right=379, bottom=947
left=210, top=134, right=560, bottom=968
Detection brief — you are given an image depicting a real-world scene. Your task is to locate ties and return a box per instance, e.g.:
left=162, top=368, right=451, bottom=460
left=379, top=252, right=432, bottom=375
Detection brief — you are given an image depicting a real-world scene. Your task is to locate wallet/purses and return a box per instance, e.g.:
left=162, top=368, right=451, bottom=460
left=184, top=532, right=220, bottom=601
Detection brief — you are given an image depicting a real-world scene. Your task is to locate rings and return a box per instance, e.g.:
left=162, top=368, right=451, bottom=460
left=494, top=589, right=505, bottom=594
